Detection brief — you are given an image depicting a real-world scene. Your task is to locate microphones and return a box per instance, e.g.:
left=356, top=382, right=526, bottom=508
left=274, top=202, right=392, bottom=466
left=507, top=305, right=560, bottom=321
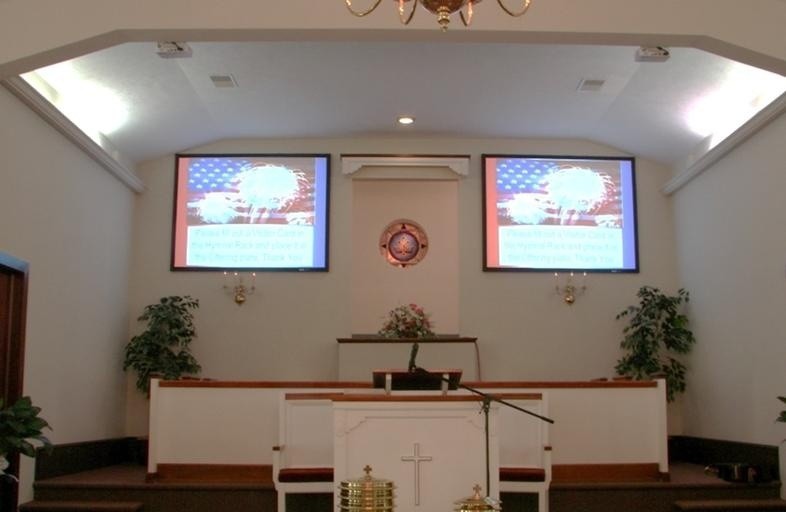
left=408, top=343, right=420, bottom=372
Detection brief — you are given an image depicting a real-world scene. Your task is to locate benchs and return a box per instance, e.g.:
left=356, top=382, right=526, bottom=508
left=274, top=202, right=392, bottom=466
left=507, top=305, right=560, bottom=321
left=272, top=391, right=548, bottom=512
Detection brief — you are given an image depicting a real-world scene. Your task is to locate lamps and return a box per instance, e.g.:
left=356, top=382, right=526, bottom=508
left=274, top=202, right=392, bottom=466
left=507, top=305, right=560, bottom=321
left=554, top=272, right=588, bottom=302
left=222, top=271, right=256, bottom=303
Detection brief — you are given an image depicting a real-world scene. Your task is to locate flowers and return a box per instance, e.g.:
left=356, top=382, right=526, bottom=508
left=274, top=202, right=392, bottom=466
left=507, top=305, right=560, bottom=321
left=377, top=302, right=440, bottom=339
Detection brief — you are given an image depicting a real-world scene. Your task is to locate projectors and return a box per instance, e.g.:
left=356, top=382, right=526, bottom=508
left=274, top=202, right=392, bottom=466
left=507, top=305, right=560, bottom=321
left=154, top=41, right=192, bottom=60
left=635, top=44, right=670, bottom=64
